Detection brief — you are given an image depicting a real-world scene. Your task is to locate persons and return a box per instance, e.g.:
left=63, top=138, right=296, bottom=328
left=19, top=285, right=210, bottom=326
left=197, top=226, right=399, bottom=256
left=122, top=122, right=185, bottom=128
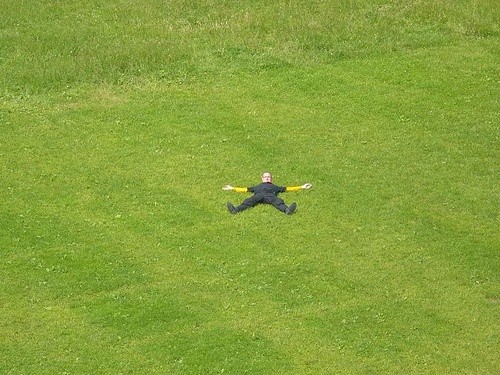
left=222, top=171, right=312, bottom=215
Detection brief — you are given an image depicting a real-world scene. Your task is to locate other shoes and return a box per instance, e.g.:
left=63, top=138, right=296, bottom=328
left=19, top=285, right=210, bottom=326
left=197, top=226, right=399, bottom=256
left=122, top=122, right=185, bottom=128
left=227, top=202, right=237, bottom=214
left=286, top=202, right=296, bottom=215
